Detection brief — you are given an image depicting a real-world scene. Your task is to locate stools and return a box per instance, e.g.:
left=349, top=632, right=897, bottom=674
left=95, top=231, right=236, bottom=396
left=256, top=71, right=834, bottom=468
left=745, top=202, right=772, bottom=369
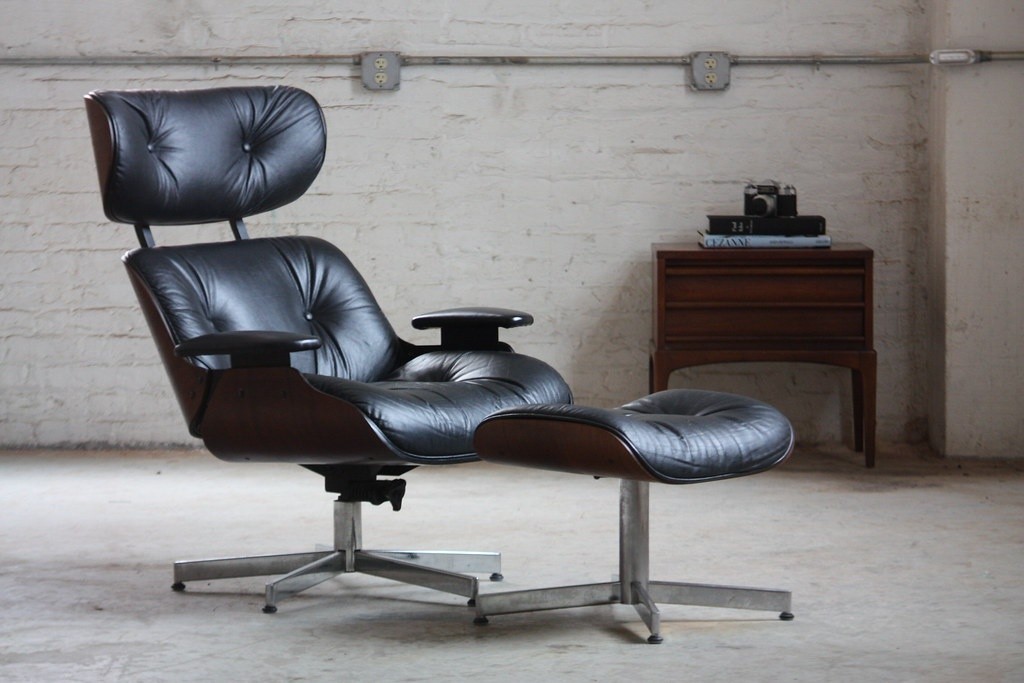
left=472, top=389, right=795, bottom=645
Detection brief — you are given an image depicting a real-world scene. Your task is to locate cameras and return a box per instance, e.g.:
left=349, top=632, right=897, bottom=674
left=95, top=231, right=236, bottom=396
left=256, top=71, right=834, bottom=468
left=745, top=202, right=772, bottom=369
left=740, top=177, right=801, bottom=219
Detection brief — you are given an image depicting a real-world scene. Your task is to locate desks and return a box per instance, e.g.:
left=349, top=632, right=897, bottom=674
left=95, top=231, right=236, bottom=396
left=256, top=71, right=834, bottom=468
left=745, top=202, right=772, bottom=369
left=649, top=241, right=877, bottom=467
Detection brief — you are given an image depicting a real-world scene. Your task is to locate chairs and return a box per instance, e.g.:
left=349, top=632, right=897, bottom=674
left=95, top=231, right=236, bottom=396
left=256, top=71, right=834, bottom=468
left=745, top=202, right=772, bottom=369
left=86, top=84, right=573, bottom=615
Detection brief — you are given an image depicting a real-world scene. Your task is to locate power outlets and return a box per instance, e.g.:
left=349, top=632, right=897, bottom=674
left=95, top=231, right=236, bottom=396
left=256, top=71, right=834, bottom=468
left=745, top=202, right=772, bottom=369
left=359, top=51, right=402, bottom=91
left=692, top=51, right=731, bottom=91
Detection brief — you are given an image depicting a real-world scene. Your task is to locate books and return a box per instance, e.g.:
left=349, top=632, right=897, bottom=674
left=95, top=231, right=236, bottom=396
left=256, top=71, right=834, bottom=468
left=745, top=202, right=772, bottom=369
left=705, top=215, right=827, bottom=235
left=697, top=229, right=832, bottom=247
